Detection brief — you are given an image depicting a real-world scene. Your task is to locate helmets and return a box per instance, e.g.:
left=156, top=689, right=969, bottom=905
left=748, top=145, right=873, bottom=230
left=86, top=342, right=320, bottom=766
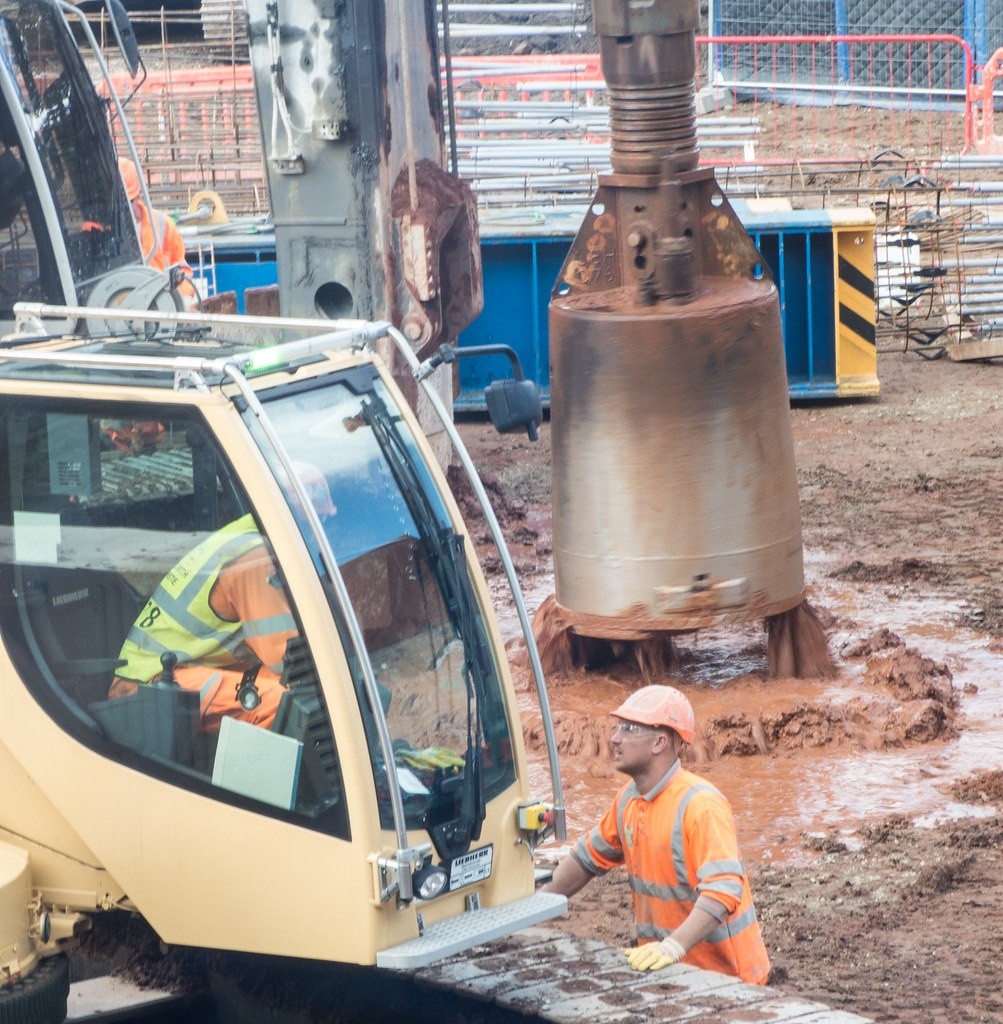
left=608, top=684, right=695, bottom=745
left=279, top=459, right=336, bottom=519
left=117, top=156, right=141, bottom=201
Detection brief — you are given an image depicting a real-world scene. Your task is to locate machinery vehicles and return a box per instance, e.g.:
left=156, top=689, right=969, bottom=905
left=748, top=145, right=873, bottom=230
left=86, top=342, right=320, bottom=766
left=0, top=294, right=884, bottom=1024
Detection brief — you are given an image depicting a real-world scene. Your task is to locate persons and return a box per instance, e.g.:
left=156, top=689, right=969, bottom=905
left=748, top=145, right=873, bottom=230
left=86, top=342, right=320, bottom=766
left=105, top=461, right=337, bottom=747
left=535, top=685, right=771, bottom=988
left=113, top=157, right=195, bottom=299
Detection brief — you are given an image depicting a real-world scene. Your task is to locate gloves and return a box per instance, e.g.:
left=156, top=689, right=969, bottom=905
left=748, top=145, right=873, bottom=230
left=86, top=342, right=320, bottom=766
left=395, top=745, right=466, bottom=771
left=624, top=937, right=686, bottom=971
